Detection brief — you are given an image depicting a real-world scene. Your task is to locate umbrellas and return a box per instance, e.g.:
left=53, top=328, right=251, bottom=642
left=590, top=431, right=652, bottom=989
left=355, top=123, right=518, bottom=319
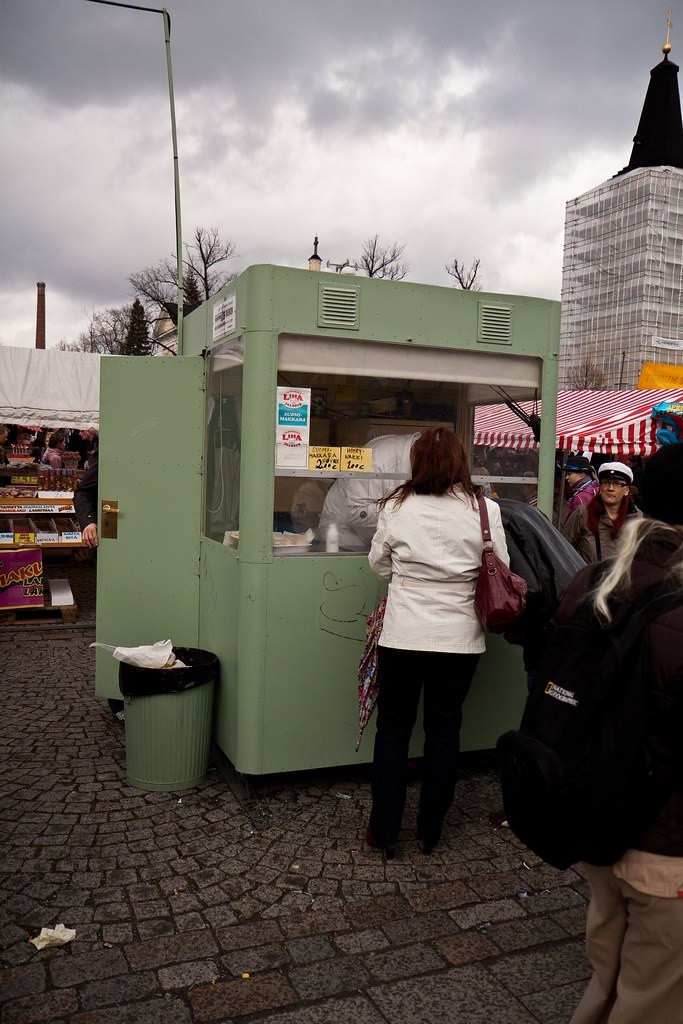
left=355, top=595, right=389, bottom=752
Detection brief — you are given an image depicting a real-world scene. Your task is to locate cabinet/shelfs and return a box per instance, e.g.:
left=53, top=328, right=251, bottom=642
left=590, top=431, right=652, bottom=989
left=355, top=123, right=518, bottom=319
left=0, top=497, right=88, bottom=548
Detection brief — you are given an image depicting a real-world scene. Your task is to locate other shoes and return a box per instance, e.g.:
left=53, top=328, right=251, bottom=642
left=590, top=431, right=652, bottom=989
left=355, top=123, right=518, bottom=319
left=491, top=809, right=510, bottom=828
left=113, top=710, right=125, bottom=723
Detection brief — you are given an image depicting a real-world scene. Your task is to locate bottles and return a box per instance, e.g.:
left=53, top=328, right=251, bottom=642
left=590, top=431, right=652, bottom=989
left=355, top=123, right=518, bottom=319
left=326, top=523, right=339, bottom=552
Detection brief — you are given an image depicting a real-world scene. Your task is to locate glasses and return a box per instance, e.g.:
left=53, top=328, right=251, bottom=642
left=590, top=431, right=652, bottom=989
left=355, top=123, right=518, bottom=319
left=599, top=480, right=630, bottom=488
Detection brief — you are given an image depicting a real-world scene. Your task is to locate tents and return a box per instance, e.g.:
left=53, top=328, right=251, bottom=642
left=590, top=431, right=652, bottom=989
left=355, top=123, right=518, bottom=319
left=0, top=346, right=116, bottom=429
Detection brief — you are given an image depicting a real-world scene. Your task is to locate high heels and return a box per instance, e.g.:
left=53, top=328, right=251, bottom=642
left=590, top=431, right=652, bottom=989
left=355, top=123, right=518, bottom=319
left=365, top=827, right=398, bottom=861
left=415, top=813, right=435, bottom=855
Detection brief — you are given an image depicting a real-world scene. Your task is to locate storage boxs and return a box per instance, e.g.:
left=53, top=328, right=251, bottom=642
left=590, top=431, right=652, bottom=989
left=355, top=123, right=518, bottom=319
left=231, top=533, right=313, bottom=554
left=34, top=491, right=74, bottom=499
left=48, top=579, right=74, bottom=607
left=55, top=517, right=82, bottom=543
left=32, top=520, right=59, bottom=544
left=11, top=518, right=35, bottom=543
left=0, top=519, right=14, bottom=544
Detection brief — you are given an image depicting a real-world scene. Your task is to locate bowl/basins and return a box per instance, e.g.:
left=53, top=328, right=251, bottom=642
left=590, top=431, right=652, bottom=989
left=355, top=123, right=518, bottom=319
left=368, top=397, right=397, bottom=415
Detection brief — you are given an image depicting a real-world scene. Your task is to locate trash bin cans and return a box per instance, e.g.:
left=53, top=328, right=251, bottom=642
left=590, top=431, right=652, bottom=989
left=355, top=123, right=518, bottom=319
left=119, top=647, right=222, bottom=791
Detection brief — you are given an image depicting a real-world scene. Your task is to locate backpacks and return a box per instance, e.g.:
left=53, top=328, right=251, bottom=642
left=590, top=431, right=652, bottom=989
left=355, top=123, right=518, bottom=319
left=496, top=558, right=683, bottom=873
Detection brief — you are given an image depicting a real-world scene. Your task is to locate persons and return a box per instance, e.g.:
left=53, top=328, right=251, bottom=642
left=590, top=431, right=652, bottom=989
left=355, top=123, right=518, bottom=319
left=366, top=427, right=510, bottom=858
left=273, top=484, right=327, bottom=536
left=551, top=442, right=683, bottom=1024
left=319, top=432, right=424, bottom=553
left=0, top=426, right=100, bottom=549
left=473, top=448, right=659, bottom=828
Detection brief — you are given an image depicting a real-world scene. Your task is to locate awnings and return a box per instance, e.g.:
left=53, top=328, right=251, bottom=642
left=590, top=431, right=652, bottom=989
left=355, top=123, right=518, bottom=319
left=477, top=387, right=683, bottom=456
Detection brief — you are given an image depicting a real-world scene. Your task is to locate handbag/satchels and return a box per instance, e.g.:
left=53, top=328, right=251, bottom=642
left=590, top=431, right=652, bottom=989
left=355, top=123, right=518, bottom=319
left=473, top=488, right=528, bottom=635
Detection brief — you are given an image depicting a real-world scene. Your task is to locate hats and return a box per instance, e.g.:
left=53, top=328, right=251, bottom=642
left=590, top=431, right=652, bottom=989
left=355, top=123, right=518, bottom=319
left=598, top=461, right=634, bottom=485
left=561, top=455, right=594, bottom=473
